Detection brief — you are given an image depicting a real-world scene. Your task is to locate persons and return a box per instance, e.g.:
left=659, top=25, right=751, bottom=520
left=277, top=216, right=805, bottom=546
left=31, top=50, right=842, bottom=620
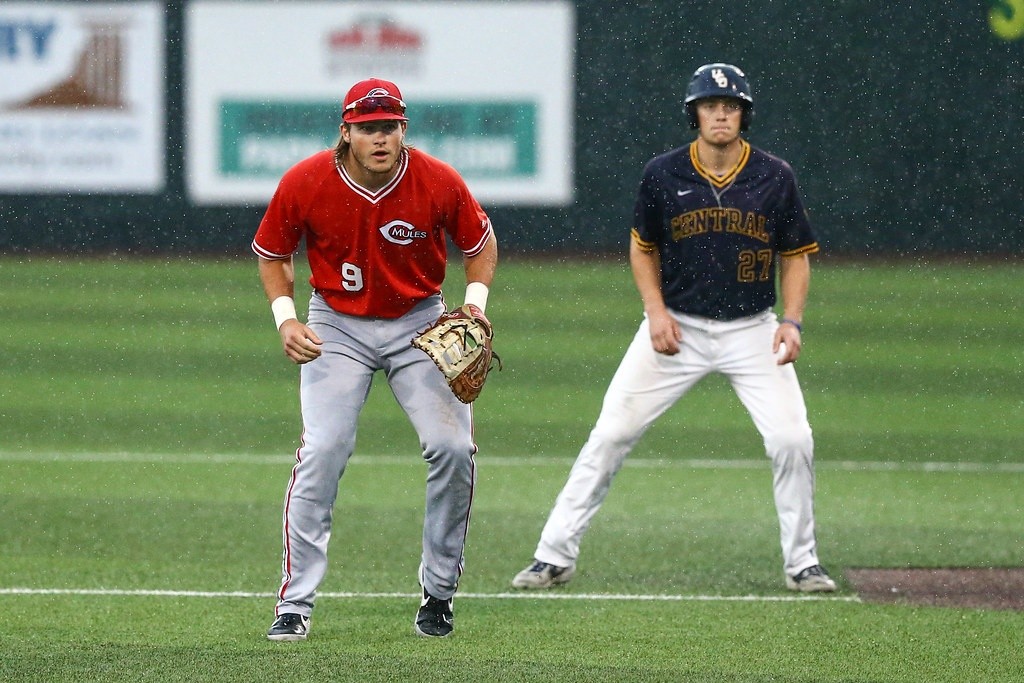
left=511, top=63, right=841, bottom=595
left=251, top=76, right=499, bottom=641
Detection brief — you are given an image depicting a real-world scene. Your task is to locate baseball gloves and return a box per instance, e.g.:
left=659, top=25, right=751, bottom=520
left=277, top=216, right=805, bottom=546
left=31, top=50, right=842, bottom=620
left=409, top=301, right=503, bottom=405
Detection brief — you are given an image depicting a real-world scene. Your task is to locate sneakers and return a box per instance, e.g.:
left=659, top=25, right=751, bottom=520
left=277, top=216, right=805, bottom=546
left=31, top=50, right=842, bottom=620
left=786, top=564, right=840, bottom=594
left=511, top=559, right=577, bottom=592
left=414, top=564, right=454, bottom=639
left=266, top=613, right=311, bottom=641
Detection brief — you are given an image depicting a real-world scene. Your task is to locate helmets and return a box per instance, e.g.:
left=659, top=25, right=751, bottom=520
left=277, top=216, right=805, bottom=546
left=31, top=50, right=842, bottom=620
left=684, top=63, right=754, bottom=132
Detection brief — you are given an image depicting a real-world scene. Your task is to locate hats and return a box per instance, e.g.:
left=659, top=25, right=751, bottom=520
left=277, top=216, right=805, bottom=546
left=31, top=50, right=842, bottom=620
left=341, top=78, right=409, bottom=124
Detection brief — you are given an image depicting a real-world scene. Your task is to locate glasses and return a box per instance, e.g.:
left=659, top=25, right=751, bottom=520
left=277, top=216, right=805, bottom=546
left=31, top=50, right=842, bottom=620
left=341, top=95, right=407, bottom=118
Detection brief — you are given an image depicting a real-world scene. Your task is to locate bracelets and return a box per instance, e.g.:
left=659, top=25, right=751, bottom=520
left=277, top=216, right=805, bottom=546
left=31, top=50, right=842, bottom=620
left=779, top=319, right=803, bottom=332
